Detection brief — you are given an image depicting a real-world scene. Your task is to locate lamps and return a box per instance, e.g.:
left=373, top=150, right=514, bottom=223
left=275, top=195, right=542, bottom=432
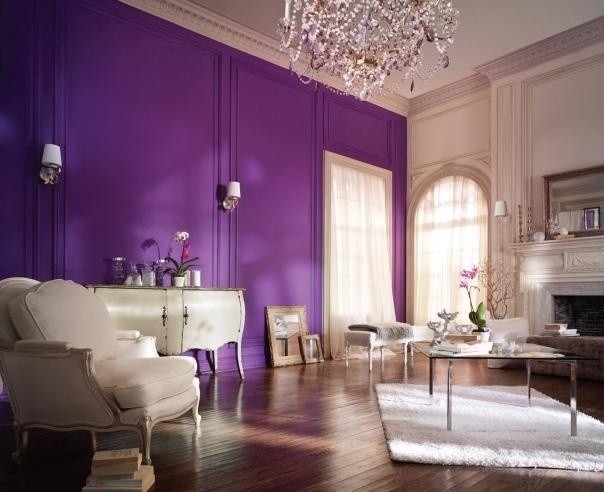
left=493, top=199, right=513, bottom=226
left=220, top=180, right=242, bottom=214
left=38, top=141, right=64, bottom=187
left=269, top=1, right=461, bottom=107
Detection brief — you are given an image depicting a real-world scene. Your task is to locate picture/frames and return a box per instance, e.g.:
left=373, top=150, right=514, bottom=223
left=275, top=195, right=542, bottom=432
left=264, top=305, right=325, bottom=367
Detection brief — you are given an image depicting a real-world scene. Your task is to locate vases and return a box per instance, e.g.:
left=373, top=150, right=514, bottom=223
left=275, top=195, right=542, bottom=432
left=171, top=271, right=185, bottom=287
left=489, top=317, right=530, bottom=350
left=476, top=328, right=490, bottom=340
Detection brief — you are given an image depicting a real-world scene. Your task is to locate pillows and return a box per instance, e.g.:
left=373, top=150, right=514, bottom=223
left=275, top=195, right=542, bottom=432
left=9, top=278, right=122, bottom=365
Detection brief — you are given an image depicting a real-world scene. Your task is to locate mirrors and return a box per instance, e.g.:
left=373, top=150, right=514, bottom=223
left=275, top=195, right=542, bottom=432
left=543, top=167, right=604, bottom=240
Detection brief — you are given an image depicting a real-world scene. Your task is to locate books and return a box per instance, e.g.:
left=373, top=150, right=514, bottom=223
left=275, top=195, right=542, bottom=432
left=430, top=332, right=493, bottom=355
left=543, top=324, right=580, bottom=336
left=80, top=449, right=156, bottom=492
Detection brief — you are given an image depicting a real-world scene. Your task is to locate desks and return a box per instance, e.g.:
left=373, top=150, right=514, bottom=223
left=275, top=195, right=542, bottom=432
left=510, top=240, right=604, bottom=364
left=523, top=333, right=604, bottom=385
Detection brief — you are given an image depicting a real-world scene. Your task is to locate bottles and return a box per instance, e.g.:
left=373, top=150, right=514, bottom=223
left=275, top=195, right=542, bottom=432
left=112, top=256, right=141, bottom=286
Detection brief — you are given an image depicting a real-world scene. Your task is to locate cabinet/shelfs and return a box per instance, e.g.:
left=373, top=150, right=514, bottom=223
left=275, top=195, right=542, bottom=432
left=80, top=283, right=247, bottom=384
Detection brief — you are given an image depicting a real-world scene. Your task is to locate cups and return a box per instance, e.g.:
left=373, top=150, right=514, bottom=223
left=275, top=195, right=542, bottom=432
left=191, top=267, right=201, bottom=287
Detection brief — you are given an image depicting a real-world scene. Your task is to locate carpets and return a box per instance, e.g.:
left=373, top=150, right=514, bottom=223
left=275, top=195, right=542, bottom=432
left=373, top=380, right=603, bottom=475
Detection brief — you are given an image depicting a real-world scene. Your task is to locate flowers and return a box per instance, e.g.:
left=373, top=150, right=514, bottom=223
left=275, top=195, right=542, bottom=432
left=162, top=231, right=202, bottom=276
left=457, top=262, right=488, bottom=330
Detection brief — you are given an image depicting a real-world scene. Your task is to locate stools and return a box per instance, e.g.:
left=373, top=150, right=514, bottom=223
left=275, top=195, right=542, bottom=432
left=342, top=321, right=414, bottom=372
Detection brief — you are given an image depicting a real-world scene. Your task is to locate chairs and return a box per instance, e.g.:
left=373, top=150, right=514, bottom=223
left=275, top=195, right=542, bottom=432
left=1, top=273, right=204, bottom=475
